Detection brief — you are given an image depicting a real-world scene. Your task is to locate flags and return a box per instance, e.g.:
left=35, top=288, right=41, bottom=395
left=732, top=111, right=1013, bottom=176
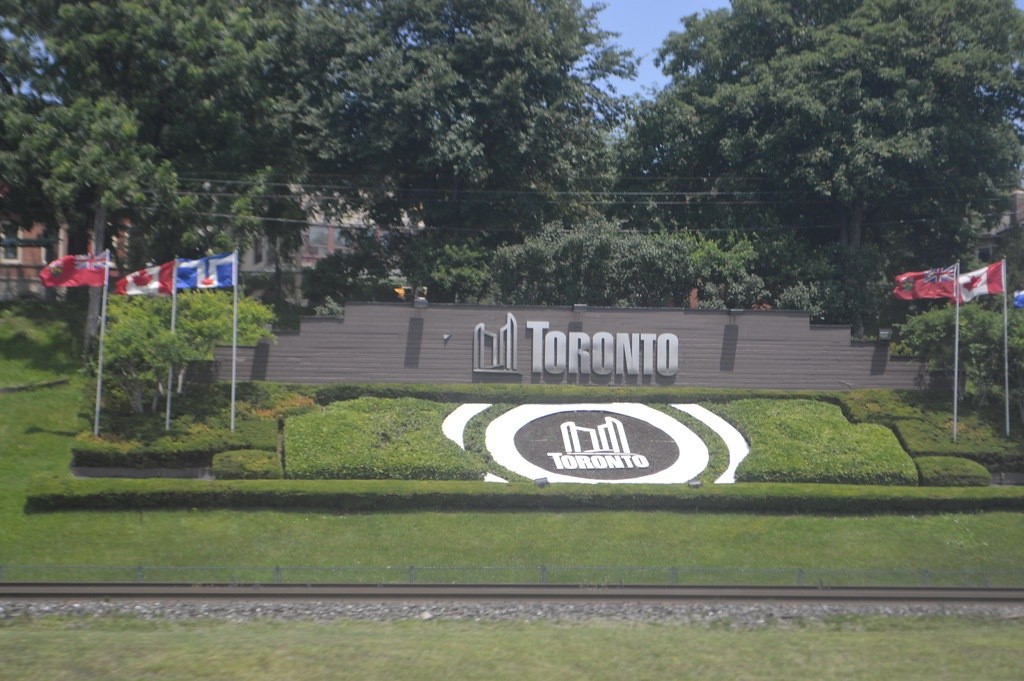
left=115, top=258, right=176, bottom=298
left=39, top=248, right=109, bottom=288
left=950, top=259, right=1003, bottom=305
left=176, top=252, right=238, bottom=288
left=892, top=262, right=956, bottom=299
left=1014, top=289, right=1024, bottom=309
